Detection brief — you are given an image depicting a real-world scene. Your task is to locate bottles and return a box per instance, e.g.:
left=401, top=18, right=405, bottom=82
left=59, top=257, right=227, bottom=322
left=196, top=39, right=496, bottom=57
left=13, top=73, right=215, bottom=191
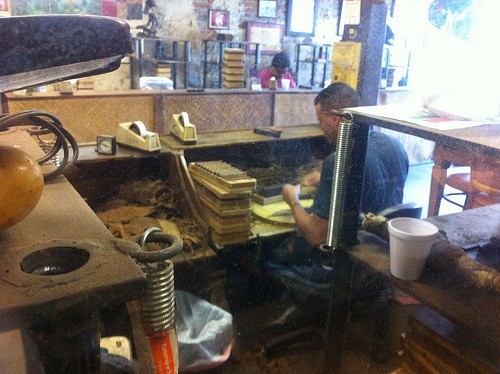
left=269, top=76, right=277, bottom=90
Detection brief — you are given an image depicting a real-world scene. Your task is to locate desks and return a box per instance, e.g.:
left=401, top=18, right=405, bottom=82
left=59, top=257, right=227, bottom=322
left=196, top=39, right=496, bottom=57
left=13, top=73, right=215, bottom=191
left=427, top=123, right=500, bottom=217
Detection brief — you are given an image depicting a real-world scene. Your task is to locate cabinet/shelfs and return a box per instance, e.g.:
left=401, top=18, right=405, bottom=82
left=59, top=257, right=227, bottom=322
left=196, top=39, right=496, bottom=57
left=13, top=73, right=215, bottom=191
left=324, top=104, right=500, bottom=374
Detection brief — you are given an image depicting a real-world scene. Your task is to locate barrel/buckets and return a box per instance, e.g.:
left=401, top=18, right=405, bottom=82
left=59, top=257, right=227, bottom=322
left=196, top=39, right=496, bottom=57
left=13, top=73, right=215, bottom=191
left=388, top=218, right=439, bottom=282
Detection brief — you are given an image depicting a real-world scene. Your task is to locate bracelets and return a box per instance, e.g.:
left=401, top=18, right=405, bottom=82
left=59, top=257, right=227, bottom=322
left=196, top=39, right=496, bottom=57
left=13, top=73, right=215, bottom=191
left=290, top=201, right=301, bottom=208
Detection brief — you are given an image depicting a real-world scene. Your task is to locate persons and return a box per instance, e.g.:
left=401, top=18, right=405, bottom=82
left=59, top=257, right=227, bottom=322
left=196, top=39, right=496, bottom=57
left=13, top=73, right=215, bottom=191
left=258, top=52, right=298, bottom=88
left=211, top=82, right=408, bottom=310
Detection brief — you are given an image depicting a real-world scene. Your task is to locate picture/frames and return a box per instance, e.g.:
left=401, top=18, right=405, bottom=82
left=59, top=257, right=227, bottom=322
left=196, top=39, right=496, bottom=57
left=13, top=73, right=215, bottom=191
left=258, top=0, right=277, bottom=18
left=209, top=10, right=229, bottom=28
left=246, top=22, right=286, bottom=55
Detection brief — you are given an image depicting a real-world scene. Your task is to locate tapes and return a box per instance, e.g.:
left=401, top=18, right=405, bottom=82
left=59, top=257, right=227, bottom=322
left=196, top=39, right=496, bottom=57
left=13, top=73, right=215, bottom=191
left=178, top=111, right=189, bottom=127
left=129, top=121, right=146, bottom=137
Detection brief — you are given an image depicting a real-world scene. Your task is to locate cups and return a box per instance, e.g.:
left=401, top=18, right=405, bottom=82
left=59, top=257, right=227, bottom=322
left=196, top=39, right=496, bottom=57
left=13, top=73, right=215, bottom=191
left=251, top=77, right=262, bottom=91
left=281, top=79, right=290, bottom=90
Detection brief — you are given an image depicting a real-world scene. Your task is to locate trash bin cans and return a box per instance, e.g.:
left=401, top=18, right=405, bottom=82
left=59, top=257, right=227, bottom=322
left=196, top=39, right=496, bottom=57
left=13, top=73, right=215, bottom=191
left=174, top=289, right=234, bottom=374
